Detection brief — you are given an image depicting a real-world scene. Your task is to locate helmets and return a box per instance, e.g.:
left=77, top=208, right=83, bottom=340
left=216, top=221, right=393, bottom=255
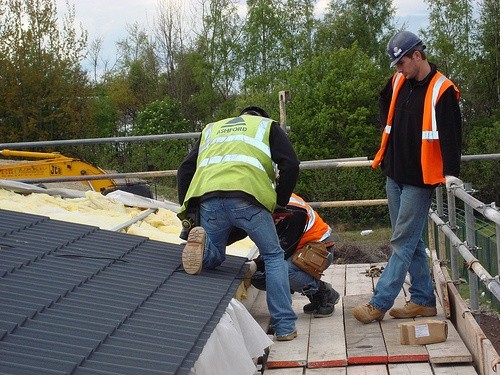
left=240, top=106, right=270, bottom=118
left=387, top=31, right=423, bottom=68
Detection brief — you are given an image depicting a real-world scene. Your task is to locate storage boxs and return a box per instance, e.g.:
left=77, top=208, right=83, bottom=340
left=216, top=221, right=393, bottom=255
left=400, top=319, right=448, bottom=346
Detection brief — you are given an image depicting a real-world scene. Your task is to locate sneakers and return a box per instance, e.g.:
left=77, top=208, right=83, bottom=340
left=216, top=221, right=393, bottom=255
left=303, top=283, right=341, bottom=318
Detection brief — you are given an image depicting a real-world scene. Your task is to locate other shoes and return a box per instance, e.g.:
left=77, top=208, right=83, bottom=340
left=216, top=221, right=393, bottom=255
left=390, top=299, right=438, bottom=318
left=182, top=226, right=206, bottom=275
left=277, top=328, right=298, bottom=341
left=352, top=302, right=385, bottom=322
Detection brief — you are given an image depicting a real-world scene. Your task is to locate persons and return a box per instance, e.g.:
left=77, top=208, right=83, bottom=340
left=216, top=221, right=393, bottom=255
left=176, top=105, right=299, bottom=341
left=226, top=191, right=340, bottom=318
left=353, top=31, right=462, bottom=324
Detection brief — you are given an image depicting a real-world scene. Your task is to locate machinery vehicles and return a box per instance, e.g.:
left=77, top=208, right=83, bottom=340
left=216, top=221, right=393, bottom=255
left=0, top=147, right=158, bottom=199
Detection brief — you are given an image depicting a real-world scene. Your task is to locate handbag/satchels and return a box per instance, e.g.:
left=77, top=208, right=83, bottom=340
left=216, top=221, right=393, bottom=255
left=292, top=241, right=331, bottom=281
left=180, top=197, right=200, bottom=239
